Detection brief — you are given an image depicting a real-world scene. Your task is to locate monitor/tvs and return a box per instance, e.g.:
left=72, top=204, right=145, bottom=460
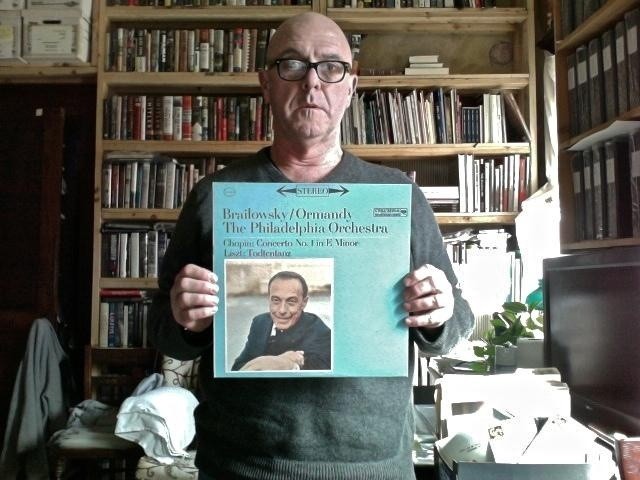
left=542, top=245, right=640, bottom=436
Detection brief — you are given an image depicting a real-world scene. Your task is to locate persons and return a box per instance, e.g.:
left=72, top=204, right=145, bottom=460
left=232, top=271, right=331, bottom=371
left=145, top=12, right=475, bottom=480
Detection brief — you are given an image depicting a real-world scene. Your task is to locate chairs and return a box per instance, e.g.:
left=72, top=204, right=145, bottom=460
left=53, top=345, right=159, bottom=480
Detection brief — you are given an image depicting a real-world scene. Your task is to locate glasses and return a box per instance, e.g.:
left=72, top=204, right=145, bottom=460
left=268, top=58, right=353, bottom=83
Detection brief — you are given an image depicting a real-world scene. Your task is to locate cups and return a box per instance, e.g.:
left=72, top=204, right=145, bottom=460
left=494, top=344, right=518, bottom=367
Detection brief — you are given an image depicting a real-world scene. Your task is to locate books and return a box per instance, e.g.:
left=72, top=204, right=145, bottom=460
left=405, top=154, right=529, bottom=213
left=340, top=87, right=529, bottom=144
left=98, top=222, right=175, bottom=348
left=404, top=55, right=449, bottom=75
left=106, top=1, right=312, bottom=72
left=102, top=93, right=275, bottom=209
left=410, top=227, right=529, bottom=387
left=343, top=31, right=362, bottom=76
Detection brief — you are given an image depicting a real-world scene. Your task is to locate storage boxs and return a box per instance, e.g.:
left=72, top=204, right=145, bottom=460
left=0, top=10, right=22, bottom=62
left=22, top=7, right=90, bottom=63
left=0, top=1, right=26, bottom=10
left=26, top=0, right=91, bottom=16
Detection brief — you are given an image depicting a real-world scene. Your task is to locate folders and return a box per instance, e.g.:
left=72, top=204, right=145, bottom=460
left=566, top=9, right=640, bottom=138
left=570, top=132, right=640, bottom=241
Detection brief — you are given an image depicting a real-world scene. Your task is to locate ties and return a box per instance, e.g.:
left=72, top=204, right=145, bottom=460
left=268, top=328, right=285, bottom=344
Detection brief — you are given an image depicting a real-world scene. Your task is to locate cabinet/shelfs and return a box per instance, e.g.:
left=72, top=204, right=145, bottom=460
left=83, top=0, right=537, bottom=404
left=552, top=0, right=639, bottom=254
